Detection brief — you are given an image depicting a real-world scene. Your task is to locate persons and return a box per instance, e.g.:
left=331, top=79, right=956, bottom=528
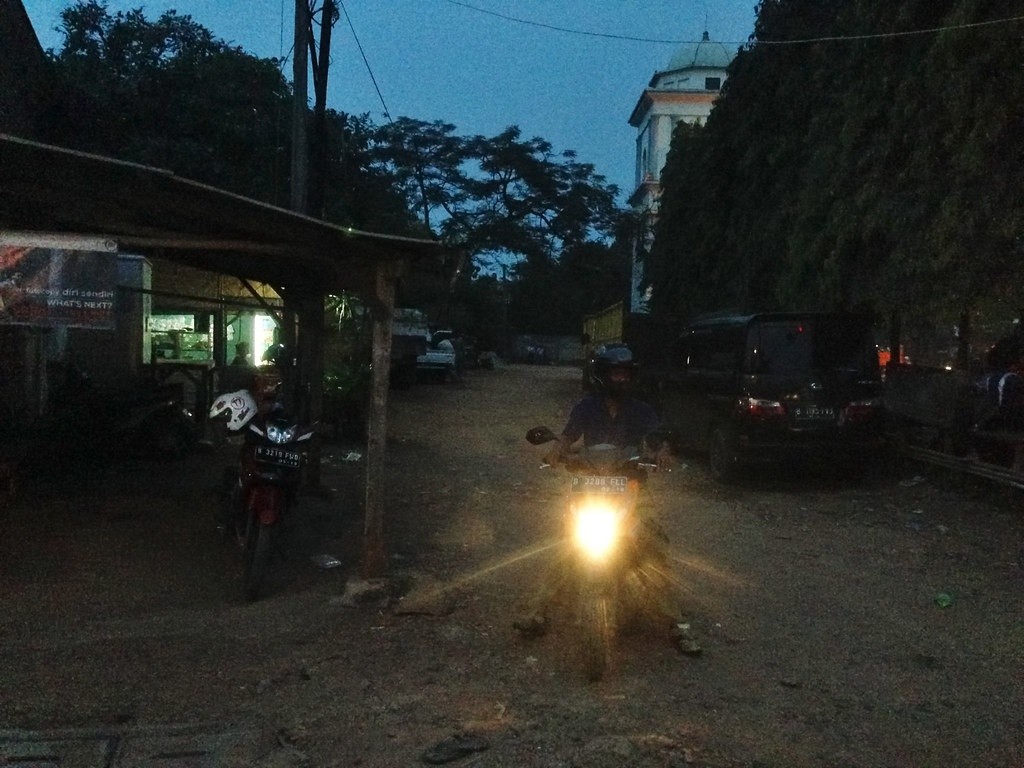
left=513, top=343, right=703, bottom=654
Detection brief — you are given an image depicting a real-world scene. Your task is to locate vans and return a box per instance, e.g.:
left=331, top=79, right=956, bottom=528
left=645, top=307, right=883, bottom=485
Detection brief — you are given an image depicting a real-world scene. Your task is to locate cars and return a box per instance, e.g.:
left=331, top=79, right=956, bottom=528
left=578, top=339, right=667, bottom=408
left=886, top=361, right=972, bottom=453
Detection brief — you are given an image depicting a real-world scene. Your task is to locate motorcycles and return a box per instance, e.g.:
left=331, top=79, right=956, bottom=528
left=221, top=411, right=325, bottom=595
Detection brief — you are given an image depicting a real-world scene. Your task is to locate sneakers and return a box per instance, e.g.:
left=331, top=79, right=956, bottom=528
left=513, top=612, right=549, bottom=634
left=669, top=618, right=702, bottom=654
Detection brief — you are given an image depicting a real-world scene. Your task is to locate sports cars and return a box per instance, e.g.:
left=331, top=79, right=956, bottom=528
left=525, top=421, right=678, bottom=687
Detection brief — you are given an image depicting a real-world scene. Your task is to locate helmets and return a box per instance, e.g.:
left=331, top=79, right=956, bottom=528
left=581, top=342, right=639, bottom=409
left=209, top=389, right=258, bottom=436
